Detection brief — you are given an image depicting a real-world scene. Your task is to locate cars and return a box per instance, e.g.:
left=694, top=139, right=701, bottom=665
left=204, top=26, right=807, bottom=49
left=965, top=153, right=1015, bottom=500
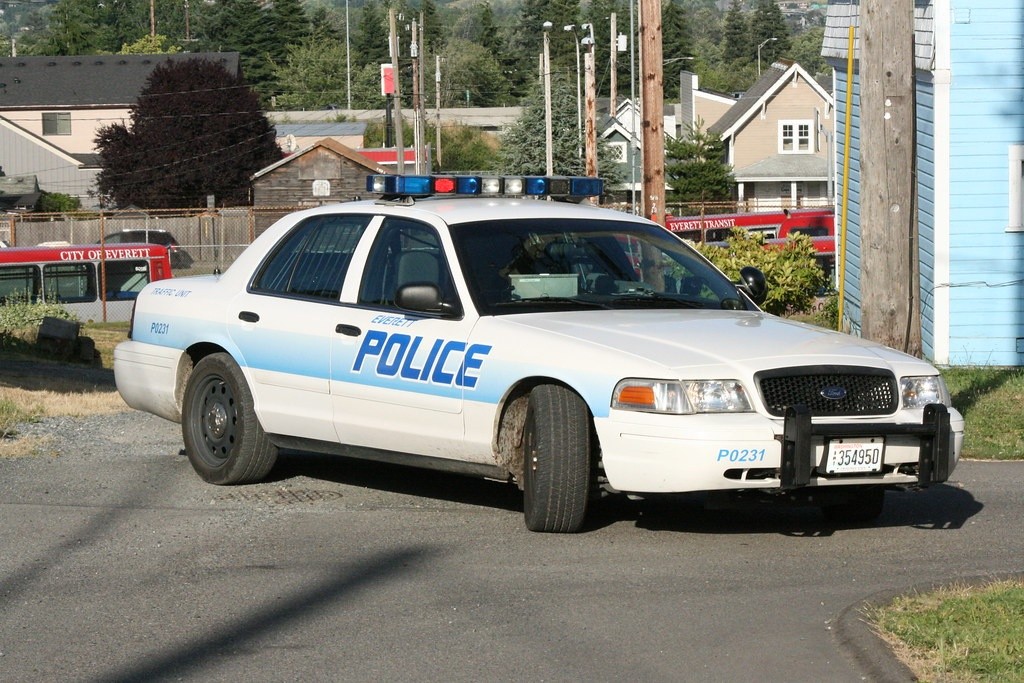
left=112, top=172, right=966, bottom=535
left=95, top=228, right=183, bottom=269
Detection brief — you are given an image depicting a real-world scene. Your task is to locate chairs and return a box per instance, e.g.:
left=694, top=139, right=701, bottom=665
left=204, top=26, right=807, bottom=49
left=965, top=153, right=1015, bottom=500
left=393, top=251, right=440, bottom=295
left=533, top=241, right=606, bottom=279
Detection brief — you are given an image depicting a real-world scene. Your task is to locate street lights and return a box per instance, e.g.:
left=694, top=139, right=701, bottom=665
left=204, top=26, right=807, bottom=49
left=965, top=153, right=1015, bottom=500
left=758, top=37, right=778, bottom=76
left=580, top=22, right=600, bottom=178
left=563, top=24, right=582, bottom=159
left=542, top=20, right=554, bottom=176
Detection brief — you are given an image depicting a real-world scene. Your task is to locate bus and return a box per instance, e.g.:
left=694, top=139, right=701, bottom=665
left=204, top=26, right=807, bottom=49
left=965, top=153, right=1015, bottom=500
left=0, top=241, right=174, bottom=326
